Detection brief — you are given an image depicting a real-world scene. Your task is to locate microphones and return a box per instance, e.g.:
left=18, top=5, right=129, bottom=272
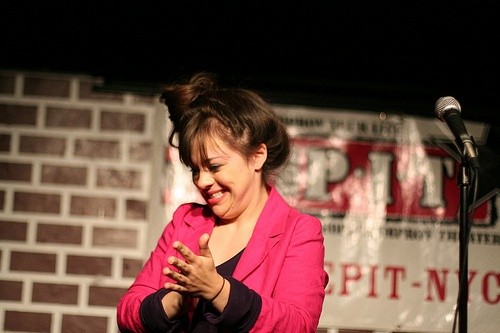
left=435, top=96, right=480, bottom=168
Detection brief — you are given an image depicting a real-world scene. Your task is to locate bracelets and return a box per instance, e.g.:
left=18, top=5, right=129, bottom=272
left=208, top=276, right=225, bottom=303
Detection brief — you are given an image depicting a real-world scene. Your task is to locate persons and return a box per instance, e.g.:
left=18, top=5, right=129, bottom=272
left=116, top=72, right=329, bottom=333
